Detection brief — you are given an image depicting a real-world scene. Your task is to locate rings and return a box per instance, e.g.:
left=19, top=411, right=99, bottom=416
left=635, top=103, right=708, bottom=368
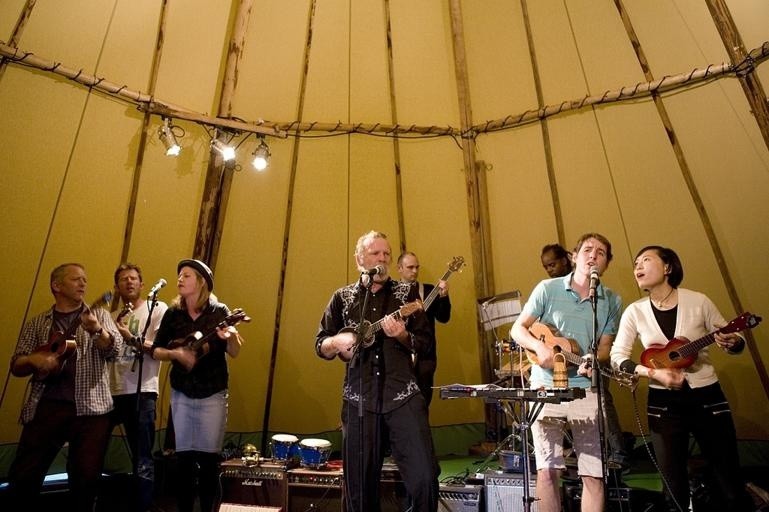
left=725, top=343, right=728, bottom=348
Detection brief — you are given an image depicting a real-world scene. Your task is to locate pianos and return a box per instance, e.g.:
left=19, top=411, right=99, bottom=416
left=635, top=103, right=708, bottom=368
left=440, top=383, right=586, bottom=404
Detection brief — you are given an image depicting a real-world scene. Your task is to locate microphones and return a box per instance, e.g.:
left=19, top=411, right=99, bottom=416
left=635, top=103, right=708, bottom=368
left=589, top=264, right=600, bottom=298
left=147, top=277, right=167, bottom=298
left=361, top=264, right=386, bottom=276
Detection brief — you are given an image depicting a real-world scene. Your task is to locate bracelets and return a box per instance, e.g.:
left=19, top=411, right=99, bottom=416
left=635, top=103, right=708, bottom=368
left=647, top=369, right=655, bottom=380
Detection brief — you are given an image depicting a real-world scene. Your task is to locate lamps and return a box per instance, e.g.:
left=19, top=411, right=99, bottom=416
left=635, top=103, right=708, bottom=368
left=202, top=122, right=235, bottom=161
left=251, top=132, right=271, bottom=171
left=159, top=117, right=180, bottom=154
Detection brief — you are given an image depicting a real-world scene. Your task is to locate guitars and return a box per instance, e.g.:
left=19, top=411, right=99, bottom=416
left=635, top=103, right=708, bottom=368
left=641, top=312, right=762, bottom=369
left=169, top=307, right=252, bottom=372
left=525, top=322, right=639, bottom=393
left=338, top=300, right=423, bottom=362
left=36, top=289, right=112, bottom=381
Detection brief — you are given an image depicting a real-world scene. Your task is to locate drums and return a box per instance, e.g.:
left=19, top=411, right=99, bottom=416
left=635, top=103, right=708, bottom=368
left=269, top=434, right=299, bottom=460
left=298, top=438, right=332, bottom=469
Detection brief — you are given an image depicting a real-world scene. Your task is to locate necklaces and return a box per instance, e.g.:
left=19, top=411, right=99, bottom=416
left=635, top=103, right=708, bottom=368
left=651, top=287, right=673, bottom=307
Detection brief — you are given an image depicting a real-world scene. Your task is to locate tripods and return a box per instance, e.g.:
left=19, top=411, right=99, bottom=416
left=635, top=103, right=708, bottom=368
left=473, top=330, right=535, bottom=476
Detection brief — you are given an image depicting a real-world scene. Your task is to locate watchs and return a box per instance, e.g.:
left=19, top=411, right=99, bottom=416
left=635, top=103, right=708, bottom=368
left=90, top=328, right=103, bottom=340
left=125, top=336, right=139, bottom=346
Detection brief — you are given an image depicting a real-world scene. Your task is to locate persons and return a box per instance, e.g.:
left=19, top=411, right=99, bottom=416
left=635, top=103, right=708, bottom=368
left=163, top=291, right=230, bottom=450
left=609, top=246, right=747, bottom=510
left=540, top=243, right=631, bottom=476
left=149, top=258, right=242, bottom=512
left=509, top=233, right=623, bottom=512
left=0, top=262, right=126, bottom=511
left=314, top=230, right=442, bottom=512
left=96, top=263, right=169, bottom=512
left=397, top=251, right=451, bottom=412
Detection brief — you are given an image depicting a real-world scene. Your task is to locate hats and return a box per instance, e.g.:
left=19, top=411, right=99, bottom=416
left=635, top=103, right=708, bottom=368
left=177, top=258, right=215, bottom=293
